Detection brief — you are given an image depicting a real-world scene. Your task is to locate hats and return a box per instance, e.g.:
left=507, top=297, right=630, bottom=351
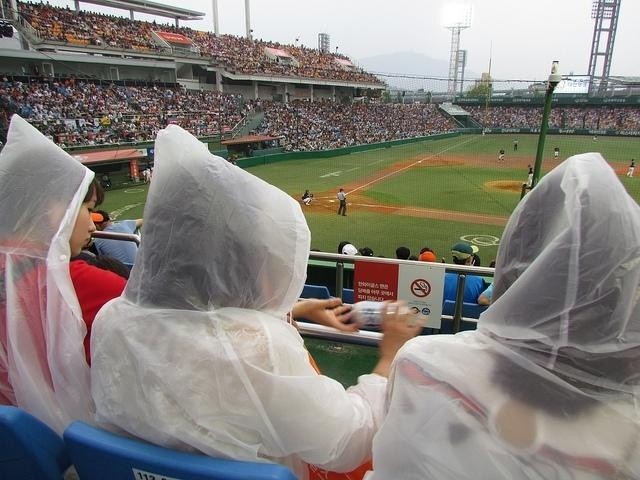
left=419, top=248, right=436, bottom=262
left=92, top=213, right=104, bottom=222
left=359, top=247, right=373, bottom=256
left=342, top=244, right=356, bottom=256
left=452, top=243, right=479, bottom=260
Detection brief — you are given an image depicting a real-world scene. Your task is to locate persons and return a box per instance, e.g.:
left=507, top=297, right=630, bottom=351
left=461, top=103, right=640, bottom=141
left=81, top=209, right=142, bottom=280
left=337, top=188, right=347, bottom=216
left=142, top=167, right=153, bottom=184
left=497, top=143, right=518, bottom=163
left=227, top=153, right=238, bottom=165
left=370, top=151, right=640, bottom=480
left=1, top=77, right=261, bottom=151
left=626, top=158, right=636, bottom=178
left=303, top=190, right=311, bottom=205
left=310, top=241, right=495, bottom=305
left=528, top=165, right=533, bottom=186
left=89, top=123, right=429, bottom=480
left=0, top=112, right=129, bottom=407
left=246, top=97, right=458, bottom=154
left=101, top=172, right=112, bottom=191
left=554, top=145, right=561, bottom=159
left=1, top=0, right=386, bottom=85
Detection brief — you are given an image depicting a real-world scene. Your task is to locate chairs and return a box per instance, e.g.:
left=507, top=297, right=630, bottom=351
left=1, top=231, right=494, bottom=479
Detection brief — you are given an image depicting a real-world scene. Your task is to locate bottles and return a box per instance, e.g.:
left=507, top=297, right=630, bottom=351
left=348, top=300, right=408, bottom=331
